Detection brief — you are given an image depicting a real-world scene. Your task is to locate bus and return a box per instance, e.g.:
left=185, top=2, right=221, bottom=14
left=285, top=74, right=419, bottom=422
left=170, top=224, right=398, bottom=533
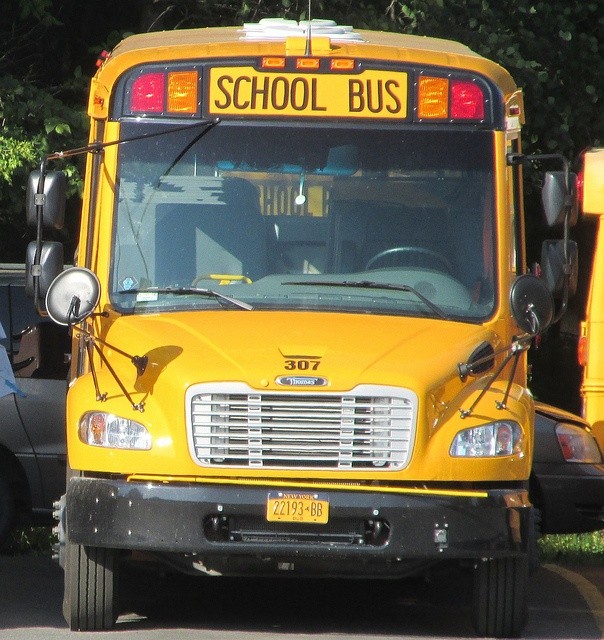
left=24, top=21, right=578, bottom=635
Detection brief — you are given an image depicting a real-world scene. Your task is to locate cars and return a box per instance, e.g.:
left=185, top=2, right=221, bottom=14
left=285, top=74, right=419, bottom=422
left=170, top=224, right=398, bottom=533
left=0, top=263, right=603, bottom=551
